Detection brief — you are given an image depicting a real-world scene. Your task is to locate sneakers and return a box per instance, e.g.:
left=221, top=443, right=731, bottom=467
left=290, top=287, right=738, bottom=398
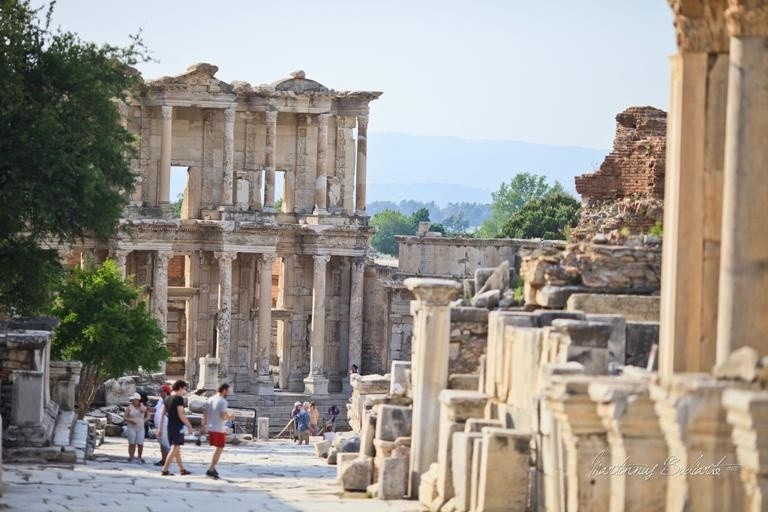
left=161, top=470, right=175, bottom=476
left=180, top=468, right=192, bottom=476
left=206, top=467, right=220, bottom=480
left=128, top=457, right=165, bottom=466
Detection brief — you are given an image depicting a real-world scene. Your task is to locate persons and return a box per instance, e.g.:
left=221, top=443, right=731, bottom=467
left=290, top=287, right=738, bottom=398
left=349, top=365, right=359, bottom=373
left=291, top=401, right=319, bottom=445
left=123, top=380, right=235, bottom=479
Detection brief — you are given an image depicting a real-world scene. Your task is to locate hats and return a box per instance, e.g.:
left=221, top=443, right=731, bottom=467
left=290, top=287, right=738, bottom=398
left=161, top=384, right=171, bottom=396
left=128, top=391, right=142, bottom=400
